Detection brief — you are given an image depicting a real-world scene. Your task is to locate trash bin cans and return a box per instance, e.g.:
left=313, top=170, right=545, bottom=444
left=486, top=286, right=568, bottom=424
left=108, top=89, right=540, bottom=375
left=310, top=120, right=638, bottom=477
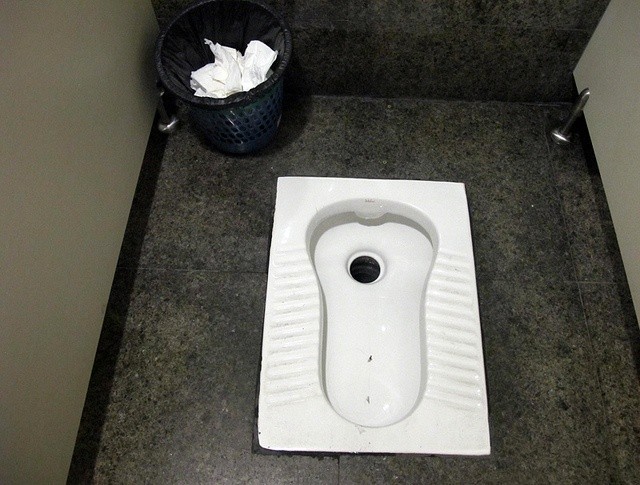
left=154, top=0, right=292, bottom=154
